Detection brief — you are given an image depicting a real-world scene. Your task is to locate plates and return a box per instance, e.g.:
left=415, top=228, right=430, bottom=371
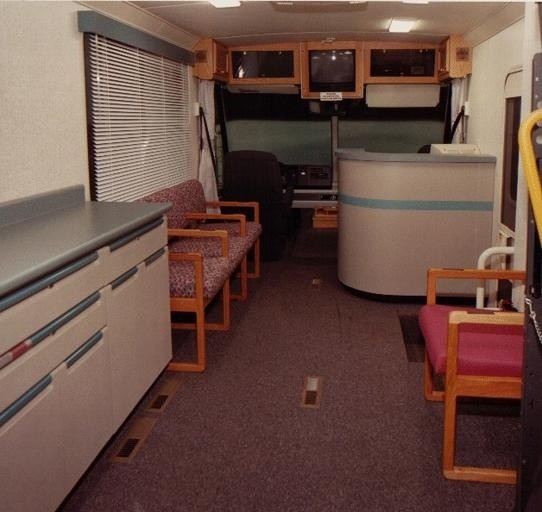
left=0, top=184, right=174, bottom=512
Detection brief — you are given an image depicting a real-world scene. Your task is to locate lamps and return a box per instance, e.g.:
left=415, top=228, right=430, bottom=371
left=130, top=180, right=263, bottom=371
left=418, top=267, right=527, bottom=485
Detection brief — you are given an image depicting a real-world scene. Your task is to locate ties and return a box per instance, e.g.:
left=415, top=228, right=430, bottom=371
left=299, top=42, right=365, bottom=99
left=430, top=143, right=483, bottom=155
